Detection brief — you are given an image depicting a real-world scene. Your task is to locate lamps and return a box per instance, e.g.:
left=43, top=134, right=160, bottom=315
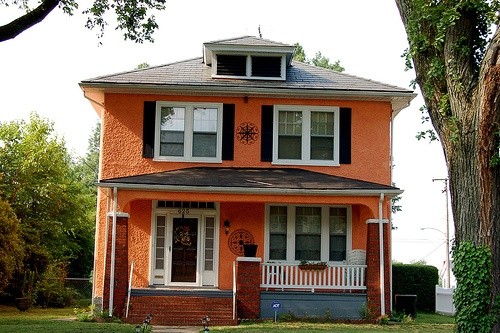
left=223, top=219, right=231, bottom=234
left=141, top=312, right=212, bottom=333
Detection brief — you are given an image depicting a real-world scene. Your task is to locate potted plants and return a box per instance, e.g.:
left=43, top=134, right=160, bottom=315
left=299, top=259, right=328, bottom=270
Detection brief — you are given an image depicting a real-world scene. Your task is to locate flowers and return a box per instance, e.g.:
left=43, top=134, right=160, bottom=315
left=174, top=224, right=193, bottom=246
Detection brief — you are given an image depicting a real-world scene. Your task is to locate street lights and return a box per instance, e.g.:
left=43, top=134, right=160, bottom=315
left=421, top=227, right=450, bottom=288
